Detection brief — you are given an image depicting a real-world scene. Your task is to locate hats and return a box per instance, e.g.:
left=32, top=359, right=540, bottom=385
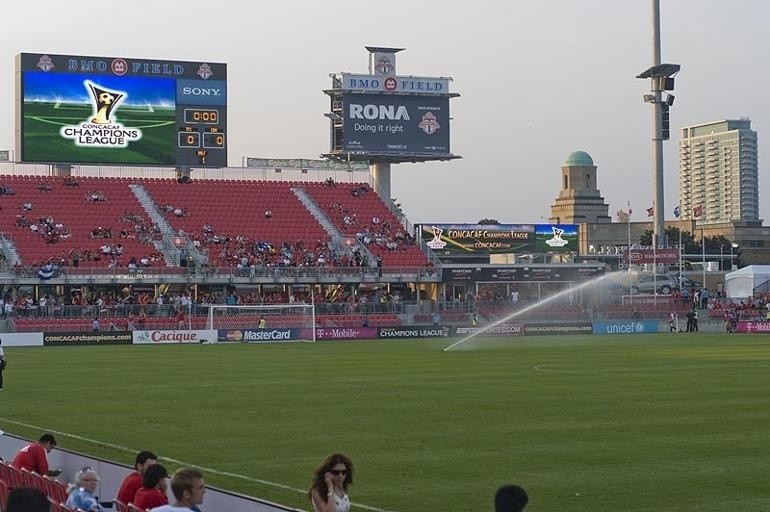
left=145, top=464, right=171, bottom=479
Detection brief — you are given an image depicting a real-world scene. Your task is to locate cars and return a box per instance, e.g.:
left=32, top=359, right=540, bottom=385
left=625, top=273, right=677, bottom=295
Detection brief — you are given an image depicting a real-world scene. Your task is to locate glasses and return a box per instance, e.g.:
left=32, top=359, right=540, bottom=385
left=81, top=479, right=99, bottom=483
left=330, top=470, right=348, bottom=476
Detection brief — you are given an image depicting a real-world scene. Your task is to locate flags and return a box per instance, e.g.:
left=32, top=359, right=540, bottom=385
left=629, top=208, right=633, bottom=215
left=647, top=206, right=653, bottom=216
left=693, top=206, right=701, bottom=217
left=674, top=205, right=680, bottom=217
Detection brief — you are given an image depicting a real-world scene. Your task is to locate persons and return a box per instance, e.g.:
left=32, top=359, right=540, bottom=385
left=1, top=177, right=444, bottom=334
left=308, top=454, right=355, bottom=512
left=3, top=432, right=205, bottom=512
left=456, top=275, right=769, bottom=335
left=0, top=339, right=5, bottom=388
left=494, top=484, right=528, bottom=511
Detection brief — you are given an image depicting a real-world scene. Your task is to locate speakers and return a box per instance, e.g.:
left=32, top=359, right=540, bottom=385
left=664, top=78, right=674, bottom=90
left=662, top=102, right=669, bottom=138
left=666, top=94, right=674, bottom=106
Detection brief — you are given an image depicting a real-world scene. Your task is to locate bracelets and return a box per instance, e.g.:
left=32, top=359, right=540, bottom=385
left=326, top=492, right=334, bottom=497
